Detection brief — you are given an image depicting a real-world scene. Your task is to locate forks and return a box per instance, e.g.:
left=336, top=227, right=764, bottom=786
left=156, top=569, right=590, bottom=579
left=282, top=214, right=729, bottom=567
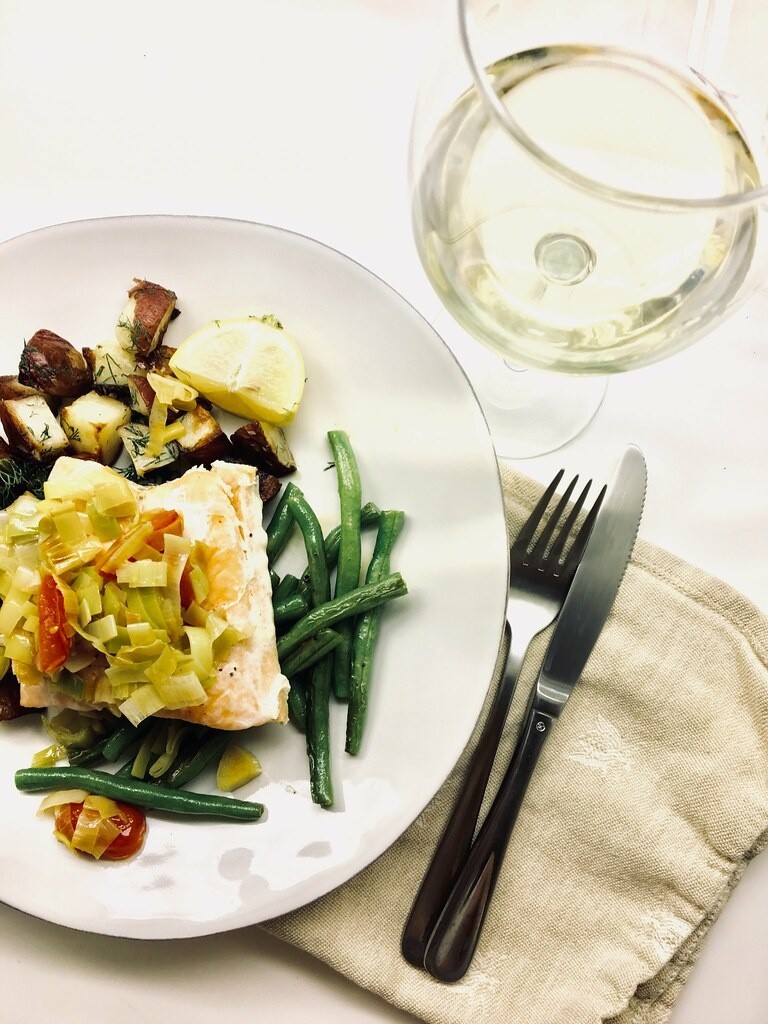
left=401, top=467, right=610, bottom=968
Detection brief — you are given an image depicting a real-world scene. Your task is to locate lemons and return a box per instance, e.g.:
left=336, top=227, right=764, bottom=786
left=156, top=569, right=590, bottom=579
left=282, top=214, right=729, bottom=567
left=164, top=314, right=305, bottom=418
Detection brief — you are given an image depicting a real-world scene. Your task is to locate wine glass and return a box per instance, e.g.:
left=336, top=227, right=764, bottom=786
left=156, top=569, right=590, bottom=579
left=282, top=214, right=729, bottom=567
left=416, top=1, right=768, bottom=460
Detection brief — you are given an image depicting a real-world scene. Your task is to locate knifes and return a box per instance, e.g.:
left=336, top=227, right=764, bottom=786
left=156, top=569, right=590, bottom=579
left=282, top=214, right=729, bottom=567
left=423, top=444, right=648, bottom=986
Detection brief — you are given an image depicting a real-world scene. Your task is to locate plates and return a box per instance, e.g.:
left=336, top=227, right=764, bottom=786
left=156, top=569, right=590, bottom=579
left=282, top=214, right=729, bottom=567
left=0, top=213, right=511, bottom=943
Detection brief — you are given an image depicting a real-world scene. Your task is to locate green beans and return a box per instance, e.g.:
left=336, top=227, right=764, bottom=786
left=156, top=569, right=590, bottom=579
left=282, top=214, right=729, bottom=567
left=14, top=423, right=407, bottom=822
